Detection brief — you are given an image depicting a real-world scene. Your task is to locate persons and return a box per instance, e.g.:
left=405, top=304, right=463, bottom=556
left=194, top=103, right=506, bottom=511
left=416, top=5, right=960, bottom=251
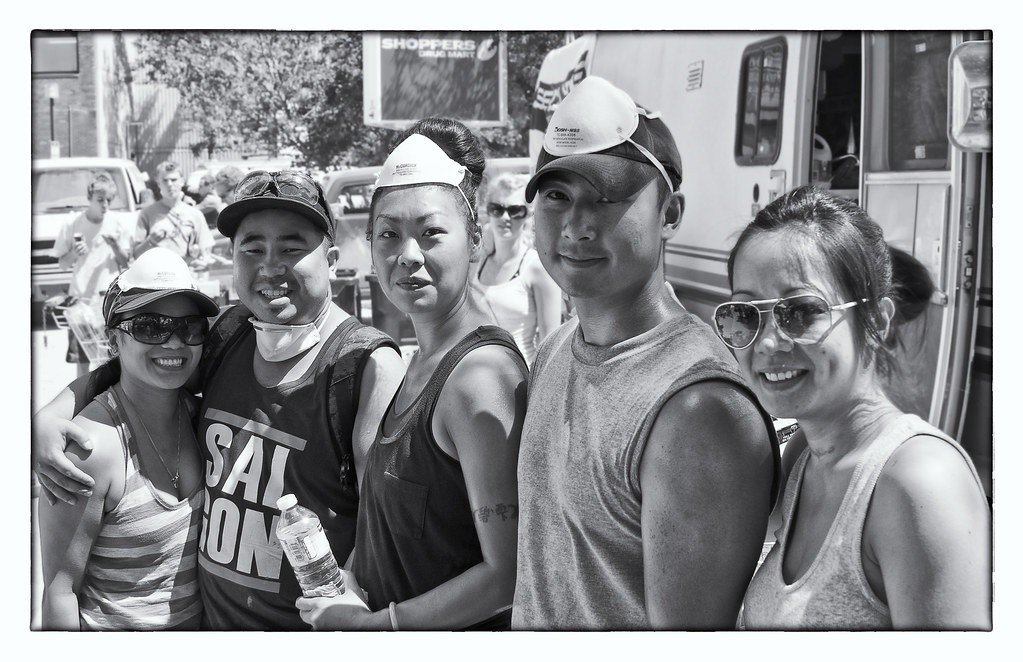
left=469, top=172, right=561, bottom=371
left=295, top=118, right=530, bottom=632
left=216, top=165, right=246, bottom=206
left=511, top=75, right=786, bottom=631
left=49, top=174, right=131, bottom=379
left=31, top=170, right=408, bottom=632
left=710, top=186, right=992, bottom=631
left=192, top=174, right=229, bottom=214
left=133, top=161, right=216, bottom=280
left=37, top=246, right=221, bottom=631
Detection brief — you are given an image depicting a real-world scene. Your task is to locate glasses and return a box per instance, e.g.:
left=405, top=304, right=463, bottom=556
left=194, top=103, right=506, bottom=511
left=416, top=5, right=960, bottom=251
left=487, top=202, right=527, bottom=219
left=712, top=294, right=871, bottom=350
left=114, top=312, right=209, bottom=345
left=234, top=170, right=319, bottom=205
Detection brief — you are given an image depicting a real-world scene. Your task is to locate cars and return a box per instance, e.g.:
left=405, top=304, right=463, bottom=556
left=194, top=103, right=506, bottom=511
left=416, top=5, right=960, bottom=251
left=321, top=167, right=385, bottom=219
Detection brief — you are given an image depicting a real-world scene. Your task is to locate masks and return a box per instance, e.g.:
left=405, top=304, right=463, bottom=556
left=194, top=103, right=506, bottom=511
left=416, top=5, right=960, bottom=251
left=543, top=75, right=639, bottom=155
left=374, top=135, right=466, bottom=187
left=118, top=248, right=197, bottom=291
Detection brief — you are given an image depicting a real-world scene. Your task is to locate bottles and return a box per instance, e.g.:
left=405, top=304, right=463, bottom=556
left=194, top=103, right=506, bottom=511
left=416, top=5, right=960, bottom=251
left=275, top=492, right=345, bottom=598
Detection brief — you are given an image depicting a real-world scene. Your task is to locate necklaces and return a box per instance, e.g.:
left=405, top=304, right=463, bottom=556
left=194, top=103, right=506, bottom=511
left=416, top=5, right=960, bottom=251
left=122, top=389, right=182, bottom=488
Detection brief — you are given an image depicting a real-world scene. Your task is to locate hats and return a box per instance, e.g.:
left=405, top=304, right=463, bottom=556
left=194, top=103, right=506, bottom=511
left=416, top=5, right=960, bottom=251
left=525, top=101, right=683, bottom=200
left=103, top=268, right=221, bottom=328
left=217, top=172, right=337, bottom=242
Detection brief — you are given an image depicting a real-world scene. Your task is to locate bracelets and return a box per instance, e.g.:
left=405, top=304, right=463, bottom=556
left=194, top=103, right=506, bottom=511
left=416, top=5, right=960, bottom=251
left=389, top=602, right=399, bottom=631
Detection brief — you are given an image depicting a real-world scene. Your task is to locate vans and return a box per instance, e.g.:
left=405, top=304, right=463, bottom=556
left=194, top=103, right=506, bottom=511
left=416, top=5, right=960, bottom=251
left=33, top=157, right=158, bottom=333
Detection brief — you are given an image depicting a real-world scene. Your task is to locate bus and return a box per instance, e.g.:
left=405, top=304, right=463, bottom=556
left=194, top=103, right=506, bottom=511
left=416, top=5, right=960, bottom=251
left=590, top=31, right=993, bottom=504
